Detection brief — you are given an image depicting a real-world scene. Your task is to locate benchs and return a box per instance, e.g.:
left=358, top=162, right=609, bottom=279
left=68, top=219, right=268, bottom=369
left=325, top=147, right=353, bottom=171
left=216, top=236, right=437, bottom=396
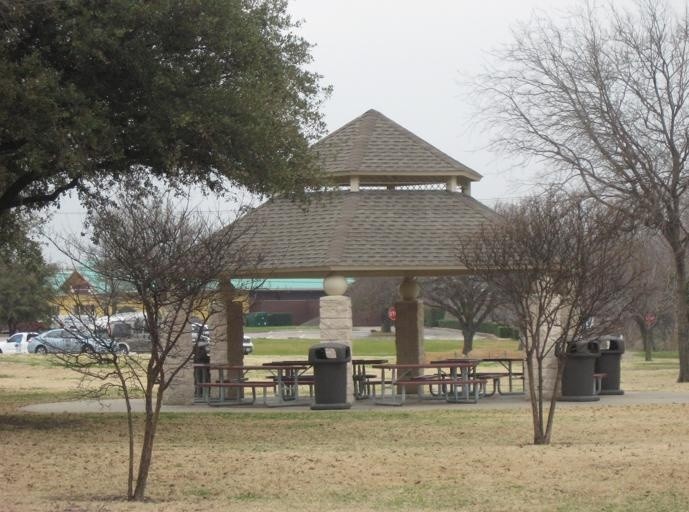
left=191, top=358, right=525, bottom=407
left=593, top=372, right=607, bottom=396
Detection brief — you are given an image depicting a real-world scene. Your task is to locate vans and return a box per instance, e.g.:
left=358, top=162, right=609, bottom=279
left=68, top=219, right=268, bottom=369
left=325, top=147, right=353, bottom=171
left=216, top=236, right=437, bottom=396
left=101, top=312, right=169, bottom=353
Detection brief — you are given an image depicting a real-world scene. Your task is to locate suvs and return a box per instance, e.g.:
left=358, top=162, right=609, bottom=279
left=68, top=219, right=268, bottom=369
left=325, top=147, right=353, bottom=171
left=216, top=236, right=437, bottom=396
left=0, top=333, right=40, bottom=354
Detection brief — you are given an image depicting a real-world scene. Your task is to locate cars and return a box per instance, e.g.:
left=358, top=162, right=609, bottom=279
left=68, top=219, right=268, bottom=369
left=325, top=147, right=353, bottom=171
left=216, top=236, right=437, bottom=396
left=53, top=313, right=98, bottom=330
left=28, top=328, right=119, bottom=354
left=243, top=335, right=254, bottom=354
left=192, top=321, right=210, bottom=355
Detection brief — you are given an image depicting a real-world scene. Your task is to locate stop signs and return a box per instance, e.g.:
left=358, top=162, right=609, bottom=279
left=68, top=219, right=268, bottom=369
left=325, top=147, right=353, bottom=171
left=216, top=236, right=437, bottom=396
left=387, top=306, right=396, bottom=321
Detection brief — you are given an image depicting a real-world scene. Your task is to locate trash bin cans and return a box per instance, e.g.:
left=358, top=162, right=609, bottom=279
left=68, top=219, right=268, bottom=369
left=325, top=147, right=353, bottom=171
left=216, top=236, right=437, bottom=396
left=554, top=333, right=626, bottom=402
left=307, top=344, right=351, bottom=410
